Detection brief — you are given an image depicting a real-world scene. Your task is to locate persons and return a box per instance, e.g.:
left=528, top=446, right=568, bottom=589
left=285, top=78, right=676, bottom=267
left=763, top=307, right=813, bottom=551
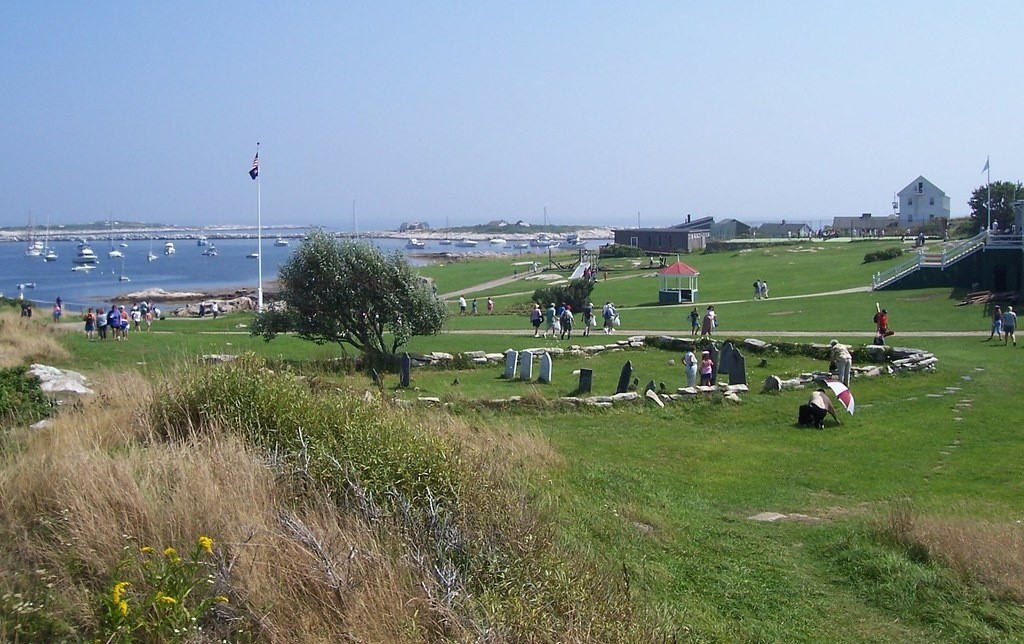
left=692, top=305, right=717, bottom=335
left=20, top=301, right=32, bottom=318
left=650, top=255, right=663, bottom=267
left=788, top=229, right=839, bottom=239
left=83, top=299, right=161, bottom=341
left=54, top=296, right=62, bottom=322
left=199, top=300, right=217, bottom=319
left=532, top=262, right=537, bottom=273
left=755, top=277, right=768, bottom=299
left=682, top=346, right=714, bottom=386
left=459, top=295, right=495, bottom=315
left=988, top=306, right=1017, bottom=346
left=981, top=219, right=1011, bottom=239
left=584, top=267, right=607, bottom=282
left=530, top=301, right=617, bottom=340
left=809, top=389, right=841, bottom=429
left=895, top=228, right=923, bottom=246
left=877, top=309, right=889, bottom=335
left=830, top=340, right=852, bottom=387
left=853, top=228, right=884, bottom=239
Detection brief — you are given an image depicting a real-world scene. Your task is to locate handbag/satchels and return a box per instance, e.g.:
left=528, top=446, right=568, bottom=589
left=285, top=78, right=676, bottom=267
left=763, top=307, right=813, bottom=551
left=885, top=328, right=894, bottom=336
left=714, top=320, right=719, bottom=327
left=874, top=336, right=885, bottom=345
left=538, top=315, right=545, bottom=322
left=612, top=314, right=620, bottom=326
left=873, top=313, right=880, bottom=323
left=590, top=316, right=597, bottom=327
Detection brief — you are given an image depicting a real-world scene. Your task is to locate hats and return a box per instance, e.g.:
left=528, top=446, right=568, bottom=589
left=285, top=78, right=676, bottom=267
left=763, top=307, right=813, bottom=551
left=551, top=303, right=555, bottom=306
left=994, top=305, right=1000, bottom=308
left=882, top=309, right=888, bottom=313
left=533, top=304, right=539, bottom=308
left=589, top=302, right=593, bottom=306
left=1008, top=306, right=1012, bottom=310
left=566, top=305, right=571, bottom=309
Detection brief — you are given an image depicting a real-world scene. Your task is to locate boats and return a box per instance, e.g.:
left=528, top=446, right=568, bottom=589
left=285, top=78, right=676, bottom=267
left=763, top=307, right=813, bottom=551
left=72, top=263, right=97, bottom=271
left=26, top=282, right=37, bottom=288
left=456, top=237, right=479, bottom=247
left=72, top=248, right=98, bottom=263
left=119, top=276, right=129, bottom=280
left=489, top=206, right=590, bottom=249
left=404, top=238, right=425, bottom=249
left=16, top=284, right=25, bottom=289
left=23, top=207, right=290, bottom=262
left=438, top=239, right=452, bottom=245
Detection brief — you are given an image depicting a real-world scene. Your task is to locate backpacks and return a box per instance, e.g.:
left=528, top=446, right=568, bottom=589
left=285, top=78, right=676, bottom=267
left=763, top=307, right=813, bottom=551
left=753, top=282, right=757, bottom=287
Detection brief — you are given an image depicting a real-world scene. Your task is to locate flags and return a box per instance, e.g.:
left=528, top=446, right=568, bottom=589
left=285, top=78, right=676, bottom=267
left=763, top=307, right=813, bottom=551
left=249, top=153, right=259, bottom=180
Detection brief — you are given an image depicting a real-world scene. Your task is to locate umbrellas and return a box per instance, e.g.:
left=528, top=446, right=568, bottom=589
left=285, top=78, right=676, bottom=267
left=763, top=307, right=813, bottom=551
left=824, top=377, right=855, bottom=415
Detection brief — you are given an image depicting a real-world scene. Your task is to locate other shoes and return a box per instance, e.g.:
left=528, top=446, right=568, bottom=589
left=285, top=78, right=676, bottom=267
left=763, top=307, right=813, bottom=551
left=535, top=334, right=540, bottom=337
left=553, top=335, right=557, bottom=337
left=543, top=333, right=546, bottom=338
left=561, top=334, right=564, bottom=339
left=587, top=334, right=588, bottom=336
left=567, top=336, right=570, bottom=338
left=583, top=332, right=586, bottom=336
